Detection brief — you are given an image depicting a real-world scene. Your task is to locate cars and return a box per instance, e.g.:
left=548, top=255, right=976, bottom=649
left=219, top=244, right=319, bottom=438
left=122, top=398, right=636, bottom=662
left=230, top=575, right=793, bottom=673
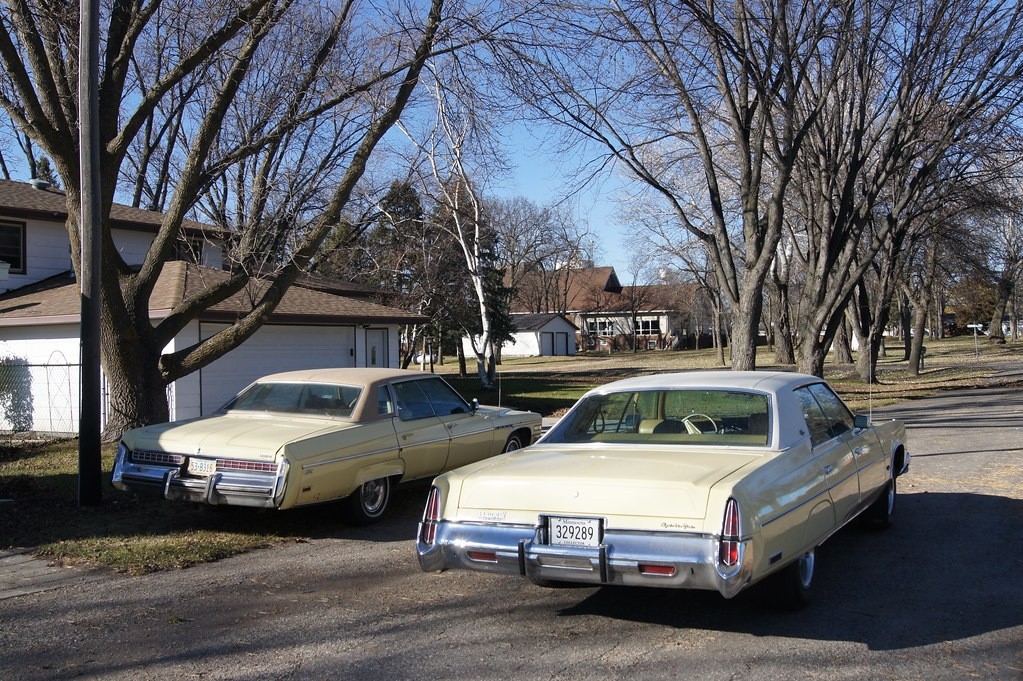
left=111, top=365, right=542, bottom=519
left=413, top=369, right=912, bottom=603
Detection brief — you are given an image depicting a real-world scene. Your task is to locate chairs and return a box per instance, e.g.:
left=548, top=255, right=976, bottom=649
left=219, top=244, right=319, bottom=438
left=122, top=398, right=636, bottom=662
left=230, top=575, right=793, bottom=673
left=748, top=413, right=769, bottom=437
left=638, top=419, right=687, bottom=435
